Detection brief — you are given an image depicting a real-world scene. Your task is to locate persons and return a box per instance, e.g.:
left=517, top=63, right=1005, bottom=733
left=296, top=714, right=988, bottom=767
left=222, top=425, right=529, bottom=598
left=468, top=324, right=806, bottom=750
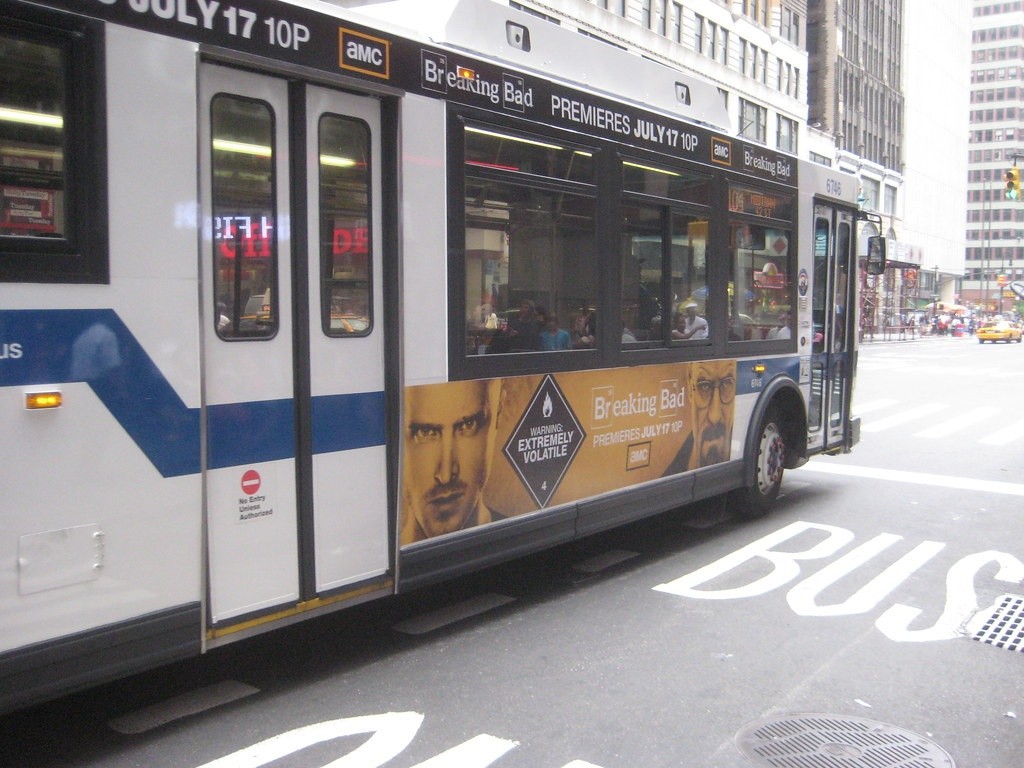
left=539, top=314, right=571, bottom=350
left=485, top=302, right=541, bottom=351
left=659, top=360, right=736, bottom=482
left=947, top=316, right=963, bottom=336
left=918, top=315, right=927, bottom=336
left=400, top=378, right=510, bottom=550
left=672, top=303, right=709, bottom=339
left=968, top=319, right=976, bottom=335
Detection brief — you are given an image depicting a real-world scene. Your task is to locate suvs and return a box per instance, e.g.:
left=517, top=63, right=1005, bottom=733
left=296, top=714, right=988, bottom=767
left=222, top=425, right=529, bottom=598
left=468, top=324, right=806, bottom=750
left=238, top=295, right=368, bottom=332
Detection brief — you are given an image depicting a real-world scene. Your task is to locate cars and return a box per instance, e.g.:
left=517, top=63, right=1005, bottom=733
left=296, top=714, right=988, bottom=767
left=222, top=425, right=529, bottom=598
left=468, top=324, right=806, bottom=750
left=691, top=285, right=763, bottom=314
left=332, top=266, right=368, bottom=283
left=764, top=287, right=840, bottom=323
left=976, top=321, right=1022, bottom=344
left=717, top=314, right=763, bottom=341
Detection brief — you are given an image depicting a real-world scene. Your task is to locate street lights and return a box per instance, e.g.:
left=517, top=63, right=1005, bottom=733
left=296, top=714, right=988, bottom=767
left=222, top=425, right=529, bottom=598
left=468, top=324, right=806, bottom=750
left=1000, top=254, right=1013, bottom=315
left=930, top=265, right=939, bottom=316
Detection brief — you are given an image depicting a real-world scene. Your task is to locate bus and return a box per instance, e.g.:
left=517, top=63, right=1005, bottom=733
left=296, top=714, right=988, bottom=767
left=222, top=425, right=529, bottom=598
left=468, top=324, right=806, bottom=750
left=0, top=0, right=886, bottom=716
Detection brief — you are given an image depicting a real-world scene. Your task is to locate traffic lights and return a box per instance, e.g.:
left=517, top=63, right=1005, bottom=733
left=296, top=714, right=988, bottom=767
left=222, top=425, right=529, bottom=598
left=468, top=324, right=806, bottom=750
left=1005, top=167, right=1021, bottom=200
